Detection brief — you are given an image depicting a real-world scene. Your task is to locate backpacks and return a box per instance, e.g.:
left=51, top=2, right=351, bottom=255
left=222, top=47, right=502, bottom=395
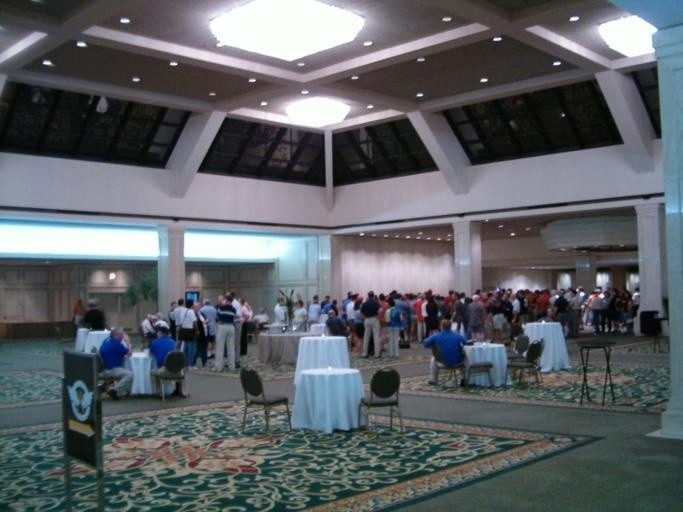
left=390, top=308, right=401, bottom=327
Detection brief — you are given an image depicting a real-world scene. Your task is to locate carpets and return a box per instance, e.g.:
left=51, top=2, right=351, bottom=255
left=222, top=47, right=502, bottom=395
left=605, top=336, right=670, bottom=357
left=362, top=352, right=674, bottom=417
left=0, top=334, right=149, bottom=357
left=0, top=368, right=64, bottom=408
left=184, top=344, right=430, bottom=383
left=0, top=395, right=605, bottom=511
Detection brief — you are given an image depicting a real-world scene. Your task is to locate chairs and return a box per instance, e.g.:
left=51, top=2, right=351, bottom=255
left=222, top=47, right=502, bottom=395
left=356, top=367, right=406, bottom=434
left=89, top=346, right=121, bottom=401
left=639, top=309, right=663, bottom=351
left=508, top=340, right=539, bottom=384
left=429, top=341, right=460, bottom=386
left=156, top=350, right=186, bottom=401
left=239, top=366, right=293, bottom=436
left=461, top=344, right=493, bottom=393
left=507, top=335, right=530, bottom=360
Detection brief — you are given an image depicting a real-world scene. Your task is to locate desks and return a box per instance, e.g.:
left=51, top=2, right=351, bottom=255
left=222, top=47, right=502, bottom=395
left=577, top=339, right=616, bottom=404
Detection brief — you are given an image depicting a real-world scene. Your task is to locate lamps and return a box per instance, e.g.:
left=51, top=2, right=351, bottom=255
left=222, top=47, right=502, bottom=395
left=598, top=14, right=659, bottom=59
left=282, top=96, right=351, bottom=128
left=208, top=1, right=365, bottom=63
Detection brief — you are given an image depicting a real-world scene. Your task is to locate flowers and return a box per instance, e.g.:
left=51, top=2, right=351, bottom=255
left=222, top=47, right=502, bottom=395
left=276, top=287, right=298, bottom=327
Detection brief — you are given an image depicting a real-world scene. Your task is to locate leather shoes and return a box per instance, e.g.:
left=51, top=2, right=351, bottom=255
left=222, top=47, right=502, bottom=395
left=427, top=379, right=437, bottom=386
left=107, top=388, right=120, bottom=401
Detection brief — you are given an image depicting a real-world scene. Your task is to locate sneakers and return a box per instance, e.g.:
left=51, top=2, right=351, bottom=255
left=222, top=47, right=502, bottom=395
left=211, top=366, right=222, bottom=372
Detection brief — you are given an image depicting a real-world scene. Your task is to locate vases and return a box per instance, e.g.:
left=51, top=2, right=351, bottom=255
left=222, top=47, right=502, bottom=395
left=287, top=314, right=293, bottom=334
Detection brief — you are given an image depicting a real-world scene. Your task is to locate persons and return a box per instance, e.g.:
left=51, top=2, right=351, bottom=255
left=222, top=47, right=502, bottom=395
left=378, top=294, right=390, bottom=310
left=384, top=297, right=404, bottom=360
left=213, top=295, right=225, bottom=322
left=275, top=297, right=289, bottom=327
left=421, top=320, right=476, bottom=389
left=393, top=294, right=410, bottom=342
left=344, top=295, right=352, bottom=321
left=309, top=295, right=322, bottom=326
left=213, top=295, right=237, bottom=372
left=401, top=295, right=408, bottom=303
left=72, top=298, right=85, bottom=330
left=322, top=300, right=336, bottom=314
left=390, top=290, right=396, bottom=296
left=352, top=299, right=364, bottom=358
left=81, top=299, right=106, bottom=331
left=324, top=310, right=350, bottom=338
left=321, top=293, right=332, bottom=309
left=240, top=299, right=254, bottom=355
left=139, top=314, right=153, bottom=338
left=167, top=302, right=178, bottom=340
left=200, top=300, right=217, bottom=358
left=346, top=294, right=359, bottom=352
left=180, top=300, right=197, bottom=370
left=193, top=301, right=210, bottom=368
left=253, top=307, right=271, bottom=325
left=342, top=291, right=352, bottom=307
left=224, top=290, right=244, bottom=367
left=152, top=312, right=170, bottom=335
left=407, top=284, right=641, bottom=342
left=98, top=328, right=134, bottom=400
left=292, top=300, right=307, bottom=331
left=361, top=291, right=383, bottom=358
left=149, top=327, right=190, bottom=399
left=174, top=299, right=185, bottom=350
